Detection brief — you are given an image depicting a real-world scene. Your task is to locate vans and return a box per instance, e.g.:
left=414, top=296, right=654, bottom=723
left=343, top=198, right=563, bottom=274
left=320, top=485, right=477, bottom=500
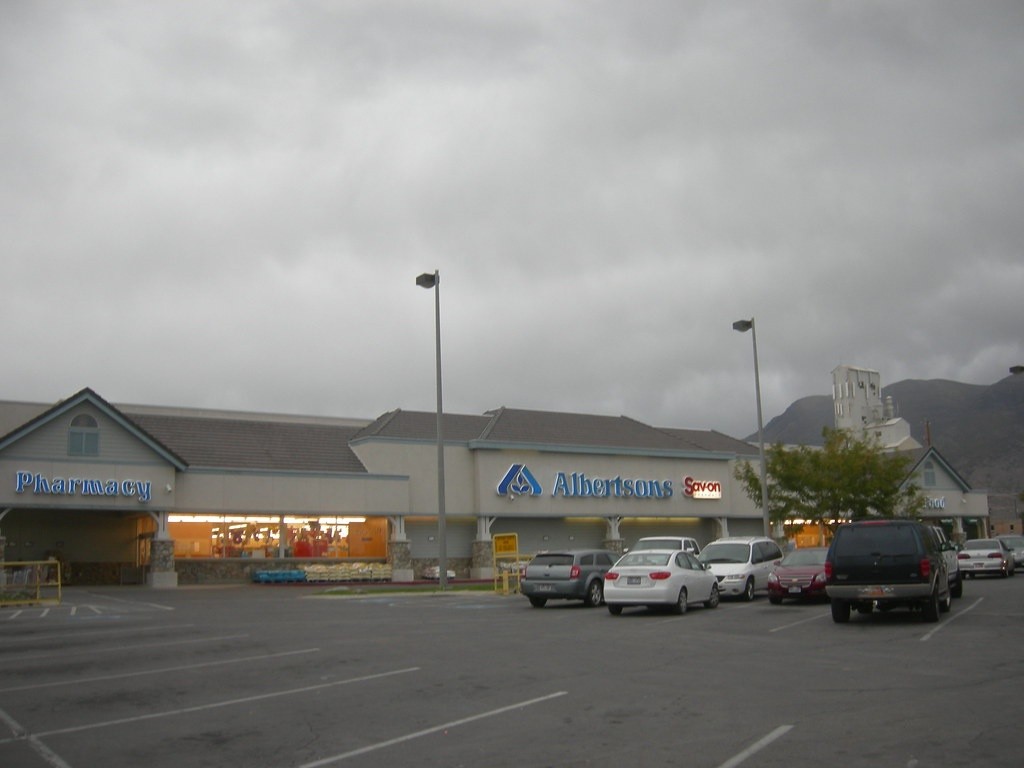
left=696, top=536, right=784, bottom=601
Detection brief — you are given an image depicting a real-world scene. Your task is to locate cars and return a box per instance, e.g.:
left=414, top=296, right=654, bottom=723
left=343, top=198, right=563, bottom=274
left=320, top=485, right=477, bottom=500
left=604, top=549, right=724, bottom=613
left=991, top=534, right=1024, bottom=571
left=958, top=538, right=1017, bottom=579
left=766, top=545, right=833, bottom=605
left=519, top=548, right=628, bottom=607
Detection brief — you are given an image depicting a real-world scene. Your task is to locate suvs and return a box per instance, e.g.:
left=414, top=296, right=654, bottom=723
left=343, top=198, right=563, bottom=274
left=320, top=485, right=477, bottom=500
left=825, top=520, right=953, bottom=623
left=621, top=535, right=701, bottom=560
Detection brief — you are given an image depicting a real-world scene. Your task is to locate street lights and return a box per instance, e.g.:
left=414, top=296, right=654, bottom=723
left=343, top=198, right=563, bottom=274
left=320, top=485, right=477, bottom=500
left=734, top=315, right=775, bottom=541
left=417, top=270, right=452, bottom=592
